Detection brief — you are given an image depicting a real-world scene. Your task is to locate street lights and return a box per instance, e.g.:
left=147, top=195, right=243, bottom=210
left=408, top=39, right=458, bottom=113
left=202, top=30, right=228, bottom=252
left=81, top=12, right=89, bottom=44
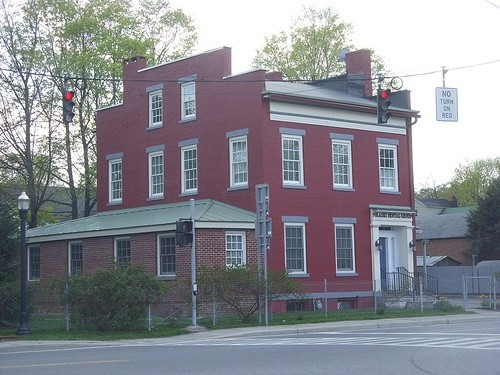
left=420, top=238, right=430, bottom=292
left=15, top=192, right=31, bottom=336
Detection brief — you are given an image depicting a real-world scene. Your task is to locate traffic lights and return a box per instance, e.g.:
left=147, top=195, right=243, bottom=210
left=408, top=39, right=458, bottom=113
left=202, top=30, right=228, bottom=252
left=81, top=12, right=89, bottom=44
left=62, top=89, right=75, bottom=123
left=377, top=88, right=391, bottom=124
left=175, top=220, right=193, bottom=246
